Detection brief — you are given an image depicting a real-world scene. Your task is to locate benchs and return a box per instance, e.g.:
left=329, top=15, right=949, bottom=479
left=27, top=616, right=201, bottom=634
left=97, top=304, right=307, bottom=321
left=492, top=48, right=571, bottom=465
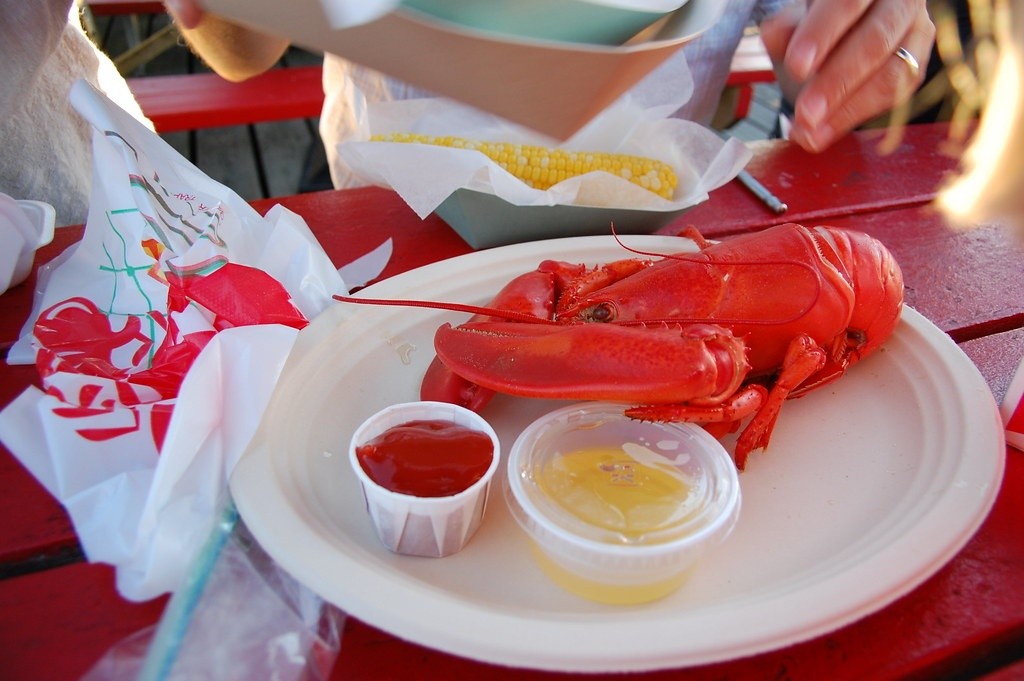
left=123, top=25, right=777, bottom=133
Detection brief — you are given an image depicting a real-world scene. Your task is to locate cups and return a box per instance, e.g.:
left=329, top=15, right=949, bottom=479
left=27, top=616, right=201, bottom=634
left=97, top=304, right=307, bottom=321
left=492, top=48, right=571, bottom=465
left=349, top=400, right=501, bottom=558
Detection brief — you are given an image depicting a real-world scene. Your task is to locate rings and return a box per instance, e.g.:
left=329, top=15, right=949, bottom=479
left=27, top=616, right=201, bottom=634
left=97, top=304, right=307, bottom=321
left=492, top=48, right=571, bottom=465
left=892, top=47, right=924, bottom=84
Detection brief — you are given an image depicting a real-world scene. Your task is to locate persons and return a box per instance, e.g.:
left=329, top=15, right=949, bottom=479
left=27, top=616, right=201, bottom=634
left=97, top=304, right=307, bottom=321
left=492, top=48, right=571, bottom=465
left=159, top=0, right=937, bottom=187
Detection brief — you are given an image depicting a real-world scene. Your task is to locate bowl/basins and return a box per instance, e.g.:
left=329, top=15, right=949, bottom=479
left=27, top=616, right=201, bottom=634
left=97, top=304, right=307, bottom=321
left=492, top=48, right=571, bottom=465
left=503, top=400, right=742, bottom=606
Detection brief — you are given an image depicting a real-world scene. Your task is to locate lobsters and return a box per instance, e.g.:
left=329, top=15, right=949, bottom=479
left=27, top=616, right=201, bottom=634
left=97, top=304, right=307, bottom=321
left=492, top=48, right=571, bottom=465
left=331, top=221, right=905, bottom=473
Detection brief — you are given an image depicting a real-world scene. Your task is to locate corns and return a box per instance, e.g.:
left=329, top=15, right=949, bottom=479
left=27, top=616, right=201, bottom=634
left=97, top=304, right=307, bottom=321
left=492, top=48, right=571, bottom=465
left=368, top=132, right=678, bottom=204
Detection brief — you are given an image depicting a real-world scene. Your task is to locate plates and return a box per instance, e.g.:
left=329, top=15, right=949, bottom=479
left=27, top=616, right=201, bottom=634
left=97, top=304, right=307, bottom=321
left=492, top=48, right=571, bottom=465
left=229, top=235, right=1006, bottom=674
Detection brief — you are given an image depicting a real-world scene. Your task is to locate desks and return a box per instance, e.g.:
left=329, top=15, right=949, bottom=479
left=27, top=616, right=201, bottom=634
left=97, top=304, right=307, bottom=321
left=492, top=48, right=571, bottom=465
left=0, top=117, right=1024, bottom=681
